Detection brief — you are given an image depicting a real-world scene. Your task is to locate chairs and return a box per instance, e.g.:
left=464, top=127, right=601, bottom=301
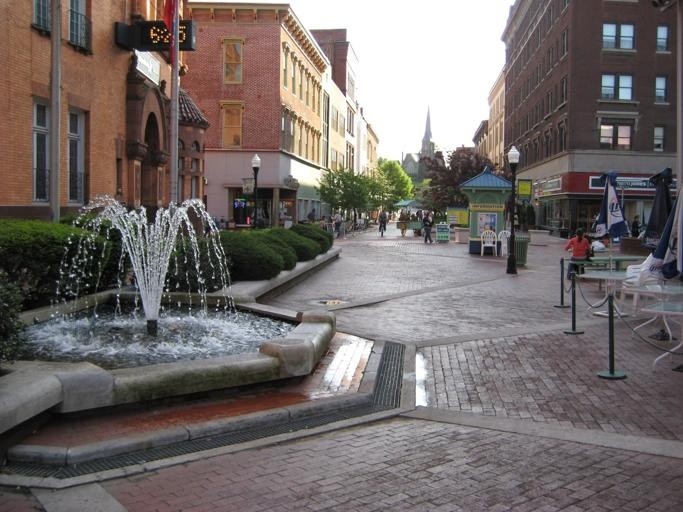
left=619, top=265, right=658, bottom=317
left=480, top=230, right=498, bottom=257
left=498, top=231, right=512, bottom=256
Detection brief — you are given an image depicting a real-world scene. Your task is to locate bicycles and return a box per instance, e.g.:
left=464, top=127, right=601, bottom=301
left=379, top=218, right=385, bottom=237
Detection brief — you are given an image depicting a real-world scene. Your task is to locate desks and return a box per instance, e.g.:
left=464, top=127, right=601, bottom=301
left=577, top=271, right=683, bottom=373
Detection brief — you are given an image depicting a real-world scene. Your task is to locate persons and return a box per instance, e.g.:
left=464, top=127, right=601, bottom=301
left=564, top=227, right=590, bottom=282
left=632, top=215, right=642, bottom=239
left=330, top=210, right=341, bottom=236
left=422, top=211, right=433, bottom=243
left=306, top=208, right=315, bottom=223
left=378, top=207, right=386, bottom=232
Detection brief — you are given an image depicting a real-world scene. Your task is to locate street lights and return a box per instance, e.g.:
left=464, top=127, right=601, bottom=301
left=506, top=145, right=519, bottom=273
left=250, top=153, right=260, bottom=228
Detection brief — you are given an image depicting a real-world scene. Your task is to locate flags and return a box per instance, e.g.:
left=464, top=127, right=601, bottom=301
left=161, top=0, right=176, bottom=69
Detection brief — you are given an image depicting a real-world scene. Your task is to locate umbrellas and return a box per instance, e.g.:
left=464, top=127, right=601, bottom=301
left=643, top=184, right=682, bottom=283
left=643, top=167, right=673, bottom=250
left=589, top=171, right=631, bottom=250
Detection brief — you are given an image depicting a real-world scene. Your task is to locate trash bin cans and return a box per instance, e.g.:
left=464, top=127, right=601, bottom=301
left=455, top=228, right=470, bottom=244
left=507, top=236, right=531, bottom=266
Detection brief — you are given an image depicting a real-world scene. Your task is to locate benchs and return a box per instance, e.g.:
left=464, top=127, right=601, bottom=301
left=569, top=256, right=648, bottom=275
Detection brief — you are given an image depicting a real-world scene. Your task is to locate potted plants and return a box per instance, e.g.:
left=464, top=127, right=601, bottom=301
left=528, top=225, right=550, bottom=245
